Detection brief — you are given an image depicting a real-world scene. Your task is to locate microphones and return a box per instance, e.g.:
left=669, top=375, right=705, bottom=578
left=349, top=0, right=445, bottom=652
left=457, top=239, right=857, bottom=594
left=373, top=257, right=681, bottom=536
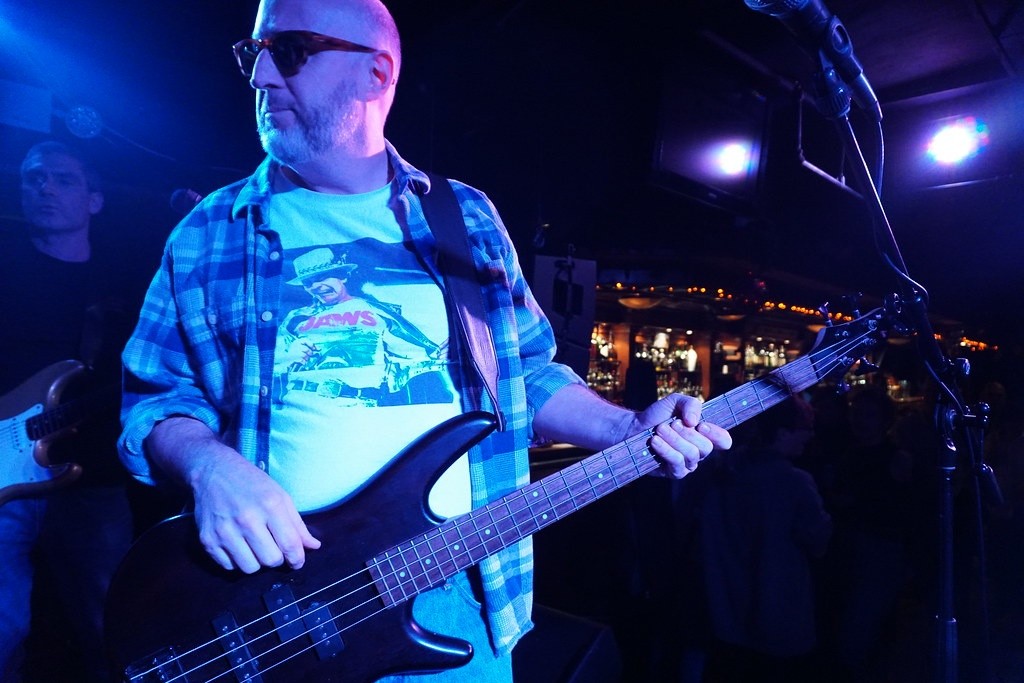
left=780, top=0, right=865, bottom=83
left=744, top=0, right=850, bottom=119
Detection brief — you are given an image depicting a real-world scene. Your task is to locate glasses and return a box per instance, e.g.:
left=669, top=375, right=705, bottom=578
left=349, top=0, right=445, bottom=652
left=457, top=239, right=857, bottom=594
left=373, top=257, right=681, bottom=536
left=234, top=29, right=379, bottom=79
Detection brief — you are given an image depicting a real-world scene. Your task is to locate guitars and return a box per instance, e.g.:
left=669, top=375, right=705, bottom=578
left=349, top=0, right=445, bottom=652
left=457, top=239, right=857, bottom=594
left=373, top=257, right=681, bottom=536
left=0, top=357, right=120, bottom=505
left=101, top=286, right=915, bottom=683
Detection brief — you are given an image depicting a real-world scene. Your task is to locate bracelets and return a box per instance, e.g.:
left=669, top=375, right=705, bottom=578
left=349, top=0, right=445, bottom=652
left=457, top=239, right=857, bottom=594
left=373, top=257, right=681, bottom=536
left=611, top=411, right=634, bottom=443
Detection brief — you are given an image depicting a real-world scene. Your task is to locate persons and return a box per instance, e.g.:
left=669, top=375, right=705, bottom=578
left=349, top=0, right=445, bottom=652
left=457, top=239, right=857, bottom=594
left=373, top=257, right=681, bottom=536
left=115, top=0, right=735, bottom=683
left=0, top=140, right=137, bottom=683
left=630, top=383, right=921, bottom=683
